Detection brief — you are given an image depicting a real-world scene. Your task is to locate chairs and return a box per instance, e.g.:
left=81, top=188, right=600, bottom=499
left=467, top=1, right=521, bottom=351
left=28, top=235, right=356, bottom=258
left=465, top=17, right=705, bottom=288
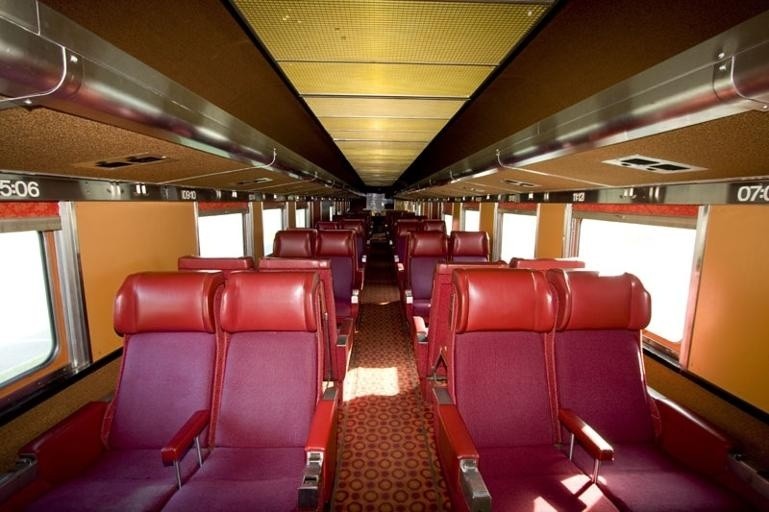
left=160, top=272, right=339, bottom=510
left=387, top=208, right=587, bottom=402
left=547, top=266, right=769, bottom=511
left=434, top=269, right=617, bottom=511
left=1, top=269, right=220, bottom=511
left=176, top=208, right=374, bottom=382
